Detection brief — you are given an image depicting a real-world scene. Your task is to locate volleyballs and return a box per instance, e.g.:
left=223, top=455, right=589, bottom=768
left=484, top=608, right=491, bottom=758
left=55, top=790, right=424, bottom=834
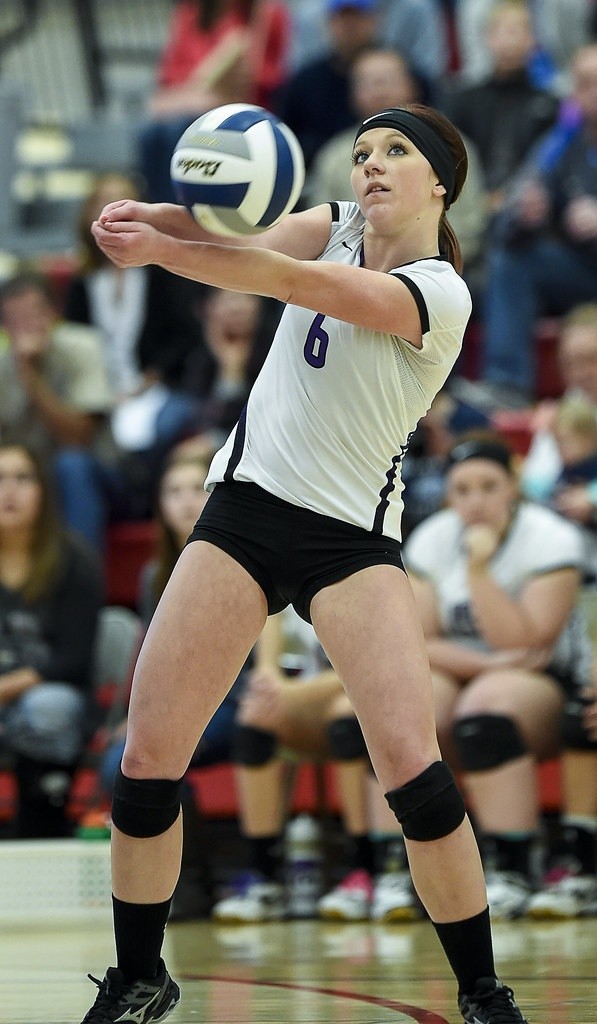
left=170, top=101, right=308, bottom=240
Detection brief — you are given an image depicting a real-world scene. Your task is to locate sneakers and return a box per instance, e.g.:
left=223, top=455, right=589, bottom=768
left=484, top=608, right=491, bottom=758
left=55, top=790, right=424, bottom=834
left=82, top=956, right=181, bottom=1024
left=458, top=976, right=529, bottom=1024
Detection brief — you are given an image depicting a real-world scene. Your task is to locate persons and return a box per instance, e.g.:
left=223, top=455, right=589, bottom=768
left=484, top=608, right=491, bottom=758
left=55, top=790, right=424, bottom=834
left=0, top=0, right=597, bottom=924
left=80, top=102, right=528, bottom=1024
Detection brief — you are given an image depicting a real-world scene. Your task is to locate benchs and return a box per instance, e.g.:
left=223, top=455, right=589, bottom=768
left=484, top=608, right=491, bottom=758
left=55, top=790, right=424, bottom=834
left=0, top=80, right=597, bottom=821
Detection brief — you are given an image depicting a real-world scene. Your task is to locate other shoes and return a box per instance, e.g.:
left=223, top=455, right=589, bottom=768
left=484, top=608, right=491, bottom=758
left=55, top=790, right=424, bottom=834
left=527, top=876, right=596, bottom=919
left=317, top=887, right=371, bottom=922
left=486, top=874, right=531, bottom=919
left=373, top=873, right=422, bottom=923
left=212, top=883, right=286, bottom=923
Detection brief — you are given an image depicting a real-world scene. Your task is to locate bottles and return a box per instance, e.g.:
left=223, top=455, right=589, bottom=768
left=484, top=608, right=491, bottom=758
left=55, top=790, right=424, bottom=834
left=77, top=807, right=114, bottom=839
left=284, top=810, right=325, bottom=914
